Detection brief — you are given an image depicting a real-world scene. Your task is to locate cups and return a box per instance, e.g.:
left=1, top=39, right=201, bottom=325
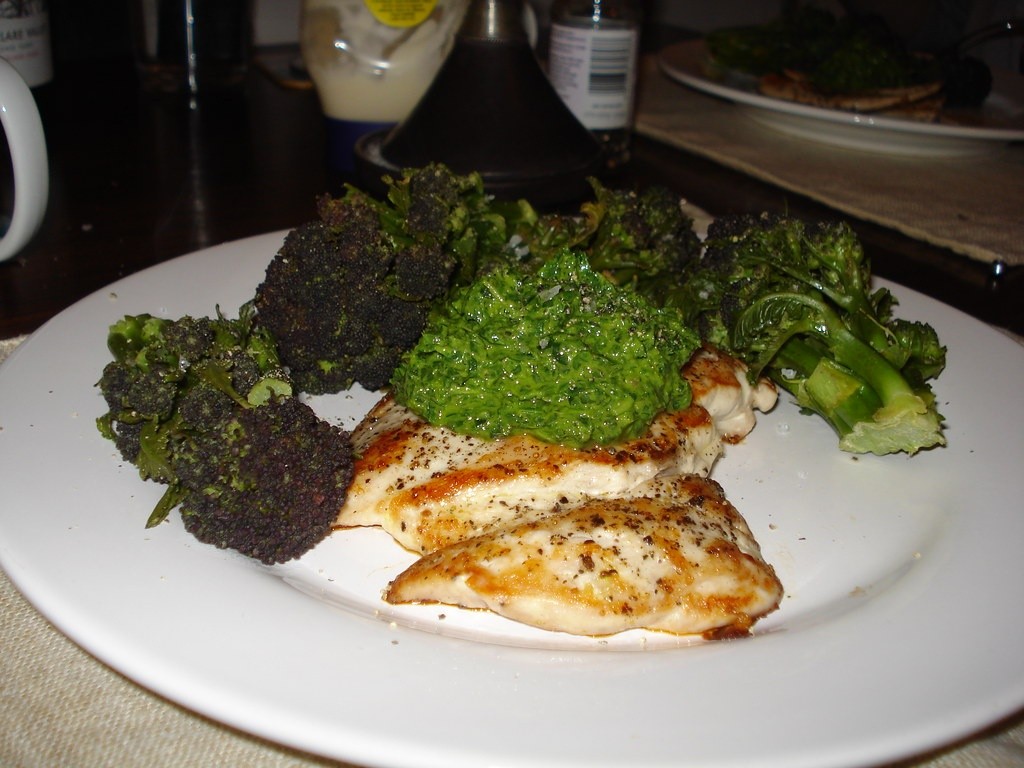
left=454, top=1, right=519, bottom=51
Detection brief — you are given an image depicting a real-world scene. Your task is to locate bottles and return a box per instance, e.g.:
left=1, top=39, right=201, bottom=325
left=0, top=1, right=57, bottom=100
left=538, top=1, right=636, bottom=163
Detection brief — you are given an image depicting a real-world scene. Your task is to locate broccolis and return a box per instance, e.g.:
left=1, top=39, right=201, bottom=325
left=92, top=163, right=948, bottom=565
left=694, top=0, right=929, bottom=96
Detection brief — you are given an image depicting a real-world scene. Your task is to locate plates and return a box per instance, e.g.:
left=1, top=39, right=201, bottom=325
left=1, top=229, right=1024, bottom=768
left=658, top=39, right=1024, bottom=153
